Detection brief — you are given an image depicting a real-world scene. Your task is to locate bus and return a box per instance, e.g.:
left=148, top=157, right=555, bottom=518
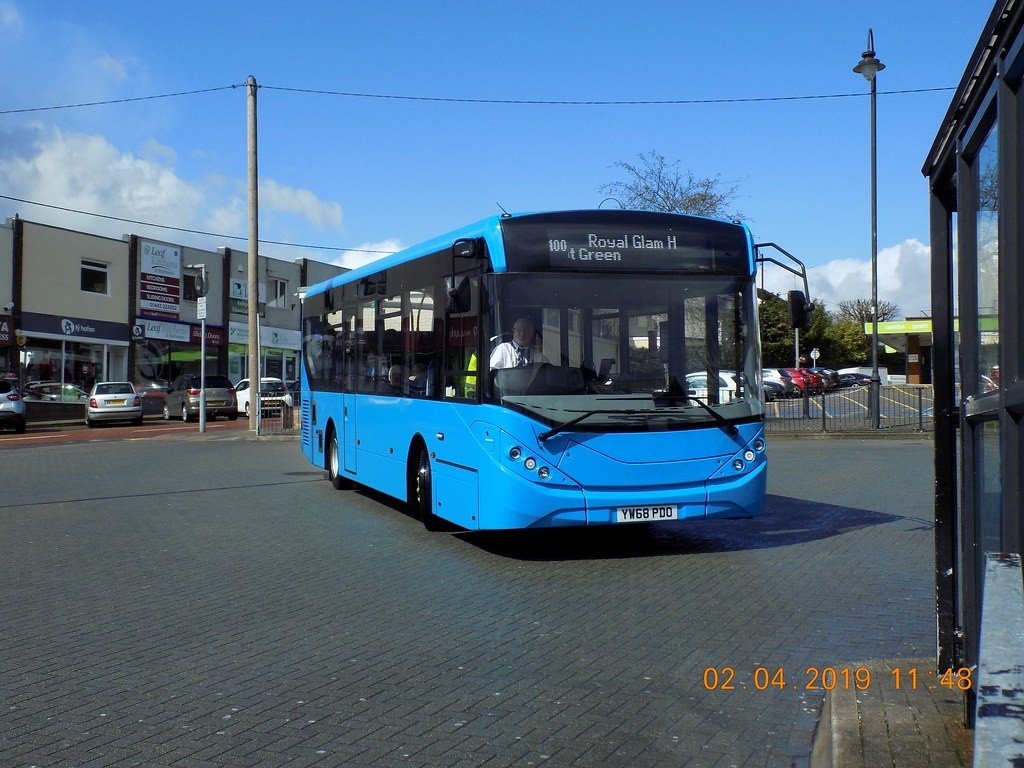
left=296, top=205, right=816, bottom=540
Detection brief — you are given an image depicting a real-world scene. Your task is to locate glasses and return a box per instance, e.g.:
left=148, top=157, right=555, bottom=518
left=513, top=327, right=530, bottom=334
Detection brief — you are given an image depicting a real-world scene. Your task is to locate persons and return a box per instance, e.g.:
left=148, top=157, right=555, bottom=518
left=414, top=363, right=425, bottom=382
left=388, top=365, right=402, bottom=386
left=490, top=318, right=550, bottom=388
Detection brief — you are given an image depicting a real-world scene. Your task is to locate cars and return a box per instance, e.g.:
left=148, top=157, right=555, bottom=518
left=653, top=365, right=889, bottom=409
left=162, top=373, right=238, bottom=423
left=135, top=377, right=174, bottom=393
left=22, top=380, right=90, bottom=405
left=83, top=378, right=144, bottom=428
left=0, top=378, right=28, bottom=435
left=233, top=376, right=293, bottom=420
left=284, top=377, right=301, bottom=408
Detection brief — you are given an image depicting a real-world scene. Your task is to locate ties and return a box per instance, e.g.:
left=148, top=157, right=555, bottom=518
left=511, top=340, right=525, bottom=368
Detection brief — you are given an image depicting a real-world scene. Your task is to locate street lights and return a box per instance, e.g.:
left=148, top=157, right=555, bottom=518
left=189, top=263, right=209, bottom=434
left=853, top=28, right=883, bottom=430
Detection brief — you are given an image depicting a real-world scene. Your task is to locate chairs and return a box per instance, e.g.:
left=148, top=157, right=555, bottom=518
left=321, top=330, right=442, bottom=397
left=120, top=388, right=125, bottom=392
left=266, top=383, right=274, bottom=387
left=100, top=388, right=107, bottom=393
